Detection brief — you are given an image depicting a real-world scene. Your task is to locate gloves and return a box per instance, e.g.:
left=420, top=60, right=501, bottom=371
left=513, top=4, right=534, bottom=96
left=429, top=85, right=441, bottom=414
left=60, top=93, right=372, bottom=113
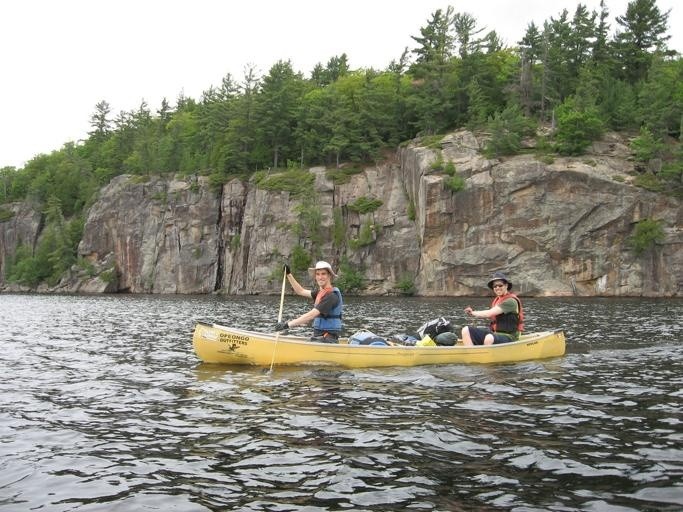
left=282, top=263, right=292, bottom=274
left=275, top=322, right=290, bottom=332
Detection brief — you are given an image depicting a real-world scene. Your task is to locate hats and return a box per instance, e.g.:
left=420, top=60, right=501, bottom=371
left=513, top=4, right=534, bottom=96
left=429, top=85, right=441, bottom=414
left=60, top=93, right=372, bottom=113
left=488, top=273, right=513, bottom=291
left=308, top=260, right=338, bottom=279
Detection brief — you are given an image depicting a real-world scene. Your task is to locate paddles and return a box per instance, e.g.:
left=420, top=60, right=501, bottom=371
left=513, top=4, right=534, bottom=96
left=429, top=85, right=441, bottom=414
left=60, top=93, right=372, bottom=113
left=271, top=264, right=287, bottom=369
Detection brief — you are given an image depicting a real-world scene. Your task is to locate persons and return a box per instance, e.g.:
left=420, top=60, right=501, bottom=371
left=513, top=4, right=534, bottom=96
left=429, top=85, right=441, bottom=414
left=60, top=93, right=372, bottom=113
left=462, top=273, right=523, bottom=346
left=274, top=261, right=343, bottom=344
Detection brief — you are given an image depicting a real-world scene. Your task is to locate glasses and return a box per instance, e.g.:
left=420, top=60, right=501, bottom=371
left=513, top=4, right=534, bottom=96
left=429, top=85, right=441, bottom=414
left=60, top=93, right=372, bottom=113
left=493, top=283, right=504, bottom=288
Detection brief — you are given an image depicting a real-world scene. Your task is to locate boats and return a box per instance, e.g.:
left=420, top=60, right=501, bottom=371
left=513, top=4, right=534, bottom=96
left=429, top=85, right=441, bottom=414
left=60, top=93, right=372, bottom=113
left=193, top=322, right=565, bottom=365
